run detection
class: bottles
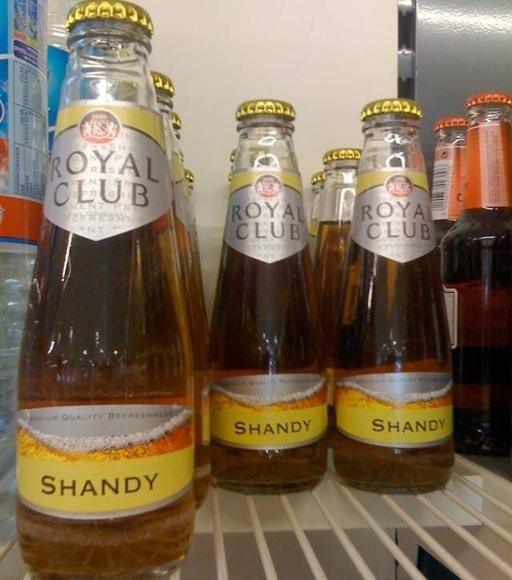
[306,171,328,263]
[148,71,211,515]
[15,1,195,580]
[437,90,512,459]
[328,98,457,495]
[207,98,332,497]
[427,117,471,244]
[309,147,362,450]
